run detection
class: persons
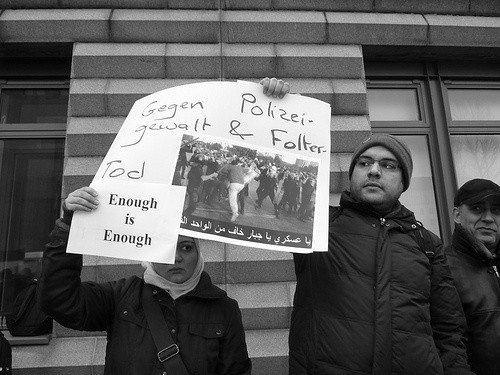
[36,186,252,375]
[172,139,316,222]
[445,179,500,375]
[259,77,471,375]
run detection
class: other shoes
[231,213,239,223]
[254,205,281,220]
[278,206,314,223]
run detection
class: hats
[453,177,500,218]
[349,131,414,192]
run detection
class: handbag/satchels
[6,255,53,336]
